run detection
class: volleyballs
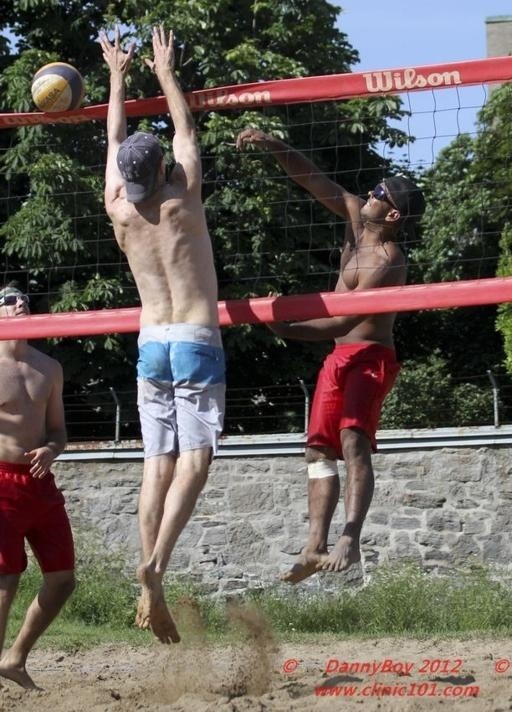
[30,61,85,114]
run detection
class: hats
[384,175,425,225]
[116,133,163,202]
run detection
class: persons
[97,26,226,645]
[0,287,75,688]
[235,129,427,585]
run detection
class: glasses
[374,185,401,211]
[0,295,30,306]
